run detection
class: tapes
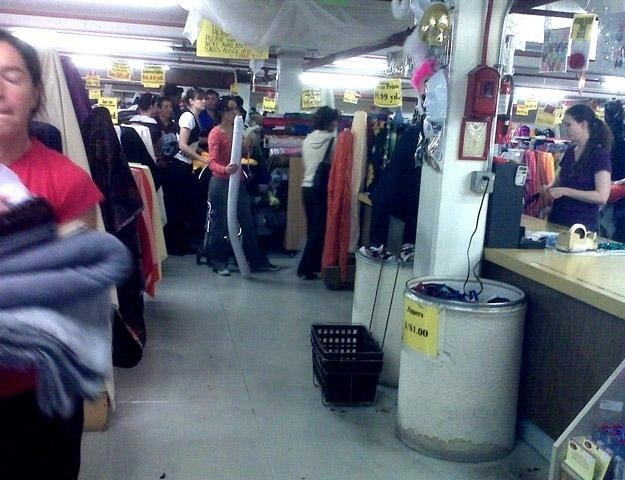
[569,222,591,238]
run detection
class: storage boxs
[310,324,384,407]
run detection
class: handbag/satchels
[159,133,179,162]
[314,137,341,192]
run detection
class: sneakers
[358,243,397,263]
[250,262,281,273]
[399,243,415,263]
[212,265,230,276]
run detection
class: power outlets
[471,170,494,194]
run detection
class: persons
[0,28,105,480]
[160,83,183,99]
[598,180,625,238]
[369,126,426,264]
[177,88,207,246]
[151,97,180,223]
[174,96,187,111]
[520,124,530,137]
[296,105,339,282]
[205,95,281,278]
[128,92,160,140]
[539,105,611,232]
[199,89,223,130]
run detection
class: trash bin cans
[397,274,526,463]
[352,244,424,391]
